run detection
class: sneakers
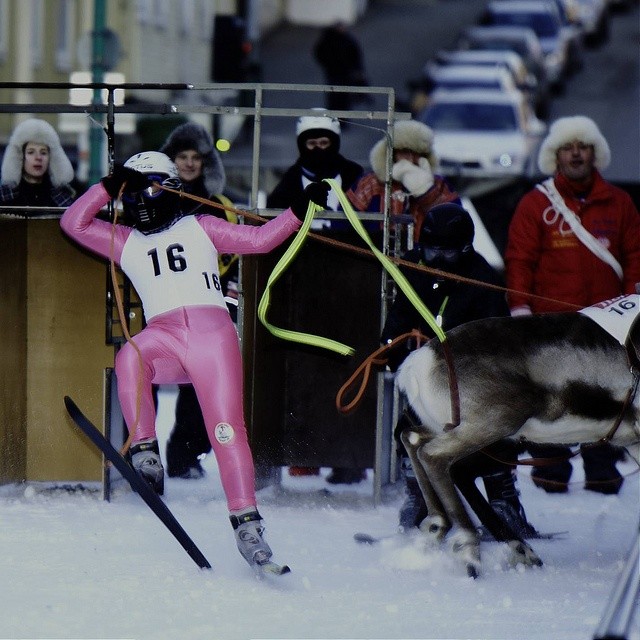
[168,441,207,480]
[289,463,323,478]
[126,438,165,497]
[326,469,368,486]
[228,506,274,567]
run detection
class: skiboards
[64,396,291,575]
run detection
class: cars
[412,0,606,178]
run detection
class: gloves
[0,180,22,205]
[48,182,77,207]
[378,334,409,364]
[99,166,154,200]
[391,158,435,199]
[289,182,332,222]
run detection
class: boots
[527,445,574,495]
[579,443,627,496]
[481,469,537,540]
[400,456,429,532]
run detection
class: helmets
[417,203,477,283]
[120,151,183,233]
[296,108,342,167]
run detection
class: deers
[392,310,640,578]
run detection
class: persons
[405,74,435,120]
[267,107,368,485]
[158,122,240,479]
[338,118,463,249]
[1,118,79,222]
[59,151,331,576]
[505,115,640,496]
[382,202,550,543]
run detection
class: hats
[160,122,226,196]
[370,120,441,184]
[539,116,612,177]
[0,118,74,188]
